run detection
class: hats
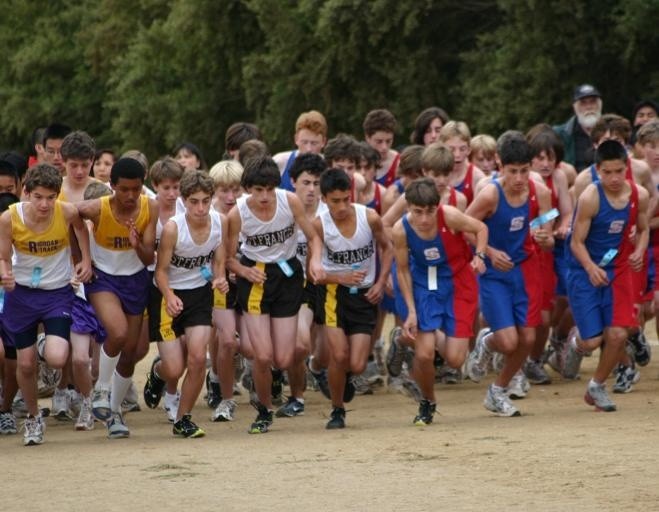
[572,85,599,101]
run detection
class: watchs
[472,252,486,260]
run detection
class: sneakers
[143,357,167,408]
[304,356,331,399]
[276,396,305,417]
[37,333,62,386]
[350,376,374,397]
[23,413,45,445]
[468,328,495,383]
[327,408,346,429]
[508,375,530,397]
[585,379,616,411]
[400,379,422,400]
[444,363,461,384]
[121,401,139,413]
[242,373,262,410]
[205,370,222,408]
[250,406,274,433]
[612,364,640,393]
[492,350,507,374]
[106,411,129,438]
[386,327,409,377]
[75,397,95,428]
[49,394,70,417]
[561,326,592,379]
[174,413,205,439]
[483,385,520,416]
[11,390,27,419]
[414,399,436,426]
[0,411,17,435]
[627,326,651,366]
[522,357,551,384]
[210,400,235,421]
[165,393,180,421]
[90,390,111,419]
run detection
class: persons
[65,156,159,439]
[154,167,232,440]
[558,138,654,414]
[390,179,488,430]
[1,84,658,441]
[1,161,96,448]
[462,135,557,417]
[305,168,394,430]
[225,154,327,436]
